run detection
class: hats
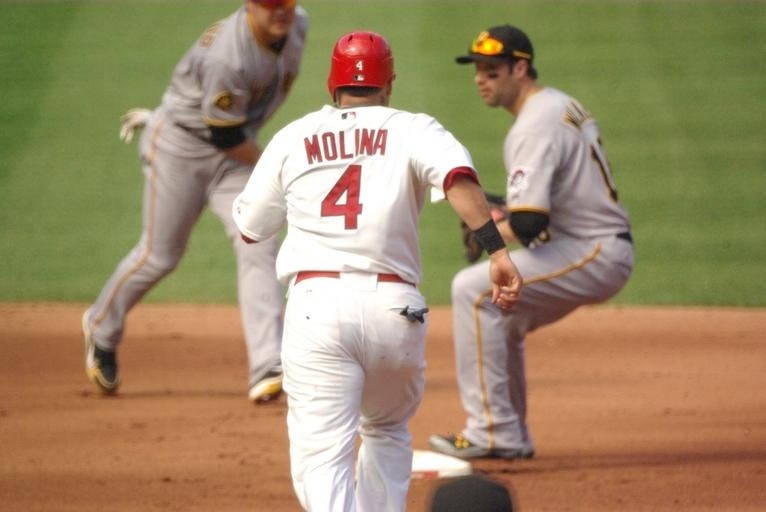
[455,25,533,65]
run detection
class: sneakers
[428,430,534,459]
[86,342,119,392]
[249,363,283,403]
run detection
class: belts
[294,271,415,286]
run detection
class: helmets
[328,32,396,103]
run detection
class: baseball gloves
[459,192,506,261]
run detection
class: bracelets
[474,217,508,257]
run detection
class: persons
[429,23,635,459]
[232,31,526,511]
[80,0,312,403]
